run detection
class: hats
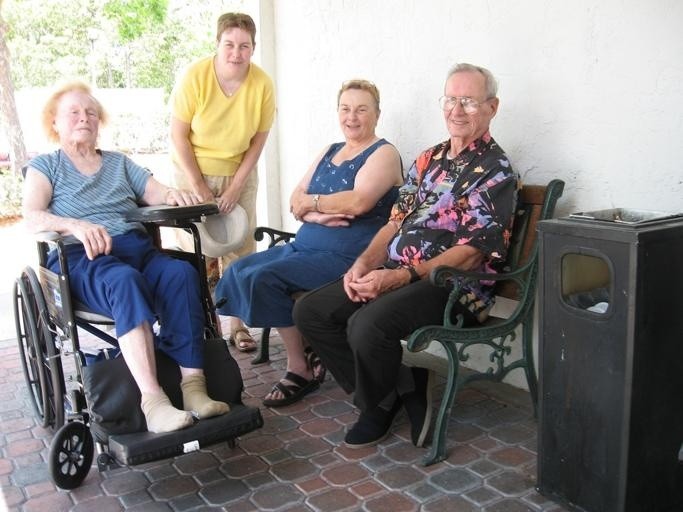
[192,197,249,258]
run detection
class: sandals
[228,326,257,352]
[263,371,319,408]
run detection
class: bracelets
[408,266,421,284]
[312,194,321,212]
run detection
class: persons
[214,80,404,408]
[21,80,230,434]
[291,62,519,448]
[171,13,277,352]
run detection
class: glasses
[438,95,495,115]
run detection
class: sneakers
[343,386,404,449]
[400,363,435,447]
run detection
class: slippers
[303,346,326,384]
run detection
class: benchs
[250,179,565,465]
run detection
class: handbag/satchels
[80,328,162,367]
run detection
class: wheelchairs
[12,200,264,491]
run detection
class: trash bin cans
[535,207,683,512]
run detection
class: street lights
[86,24,98,84]
